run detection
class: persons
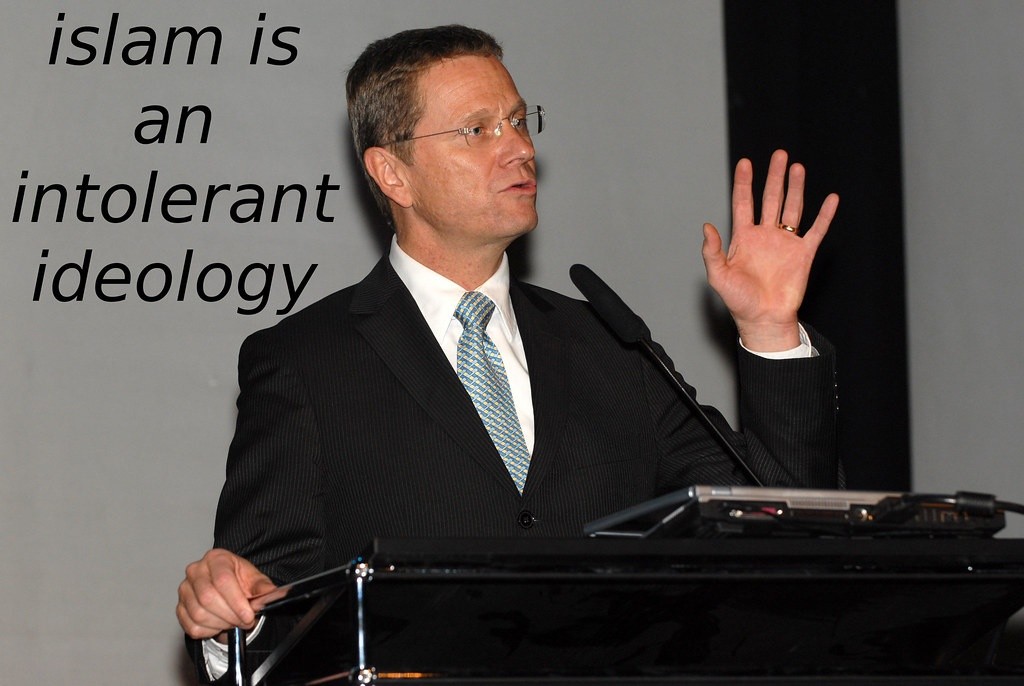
[176,24,841,683]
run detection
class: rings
[779,222,799,235]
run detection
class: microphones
[569,263,766,487]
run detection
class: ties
[452,292,531,497]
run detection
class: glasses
[376,104,549,148]
